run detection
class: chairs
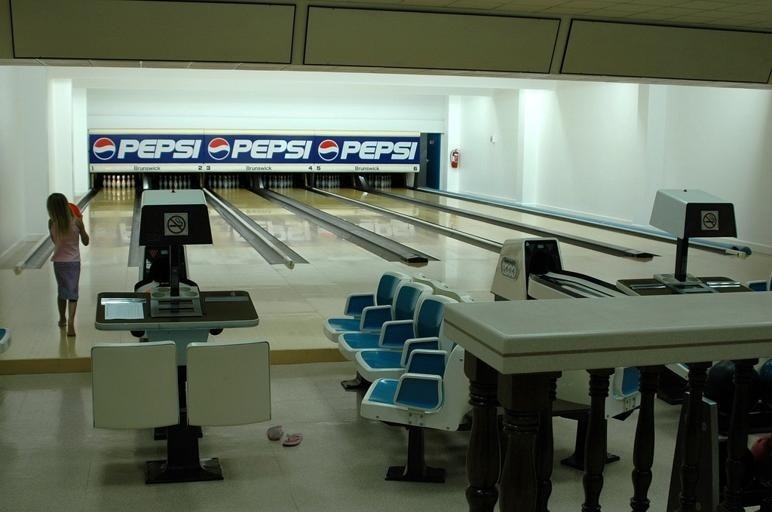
[185,342,272,481]
[360,342,474,482]
[356,295,458,384]
[90,341,177,485]
[337,280,434,361]
[322,270,412,389]
[668,393,728,510]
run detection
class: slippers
[283,432,302,446]
[267,425,283,441]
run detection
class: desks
[445,287,772,512]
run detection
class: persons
[47,193,89,337]
